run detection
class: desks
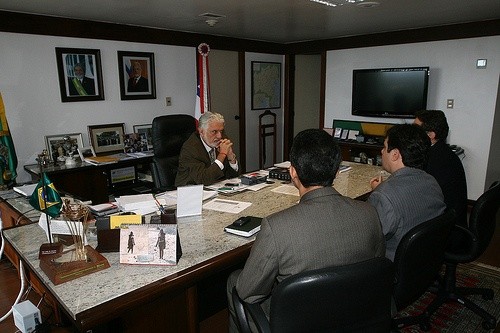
[23,151,153,206]
[0,160,391,333]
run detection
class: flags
[28,173,63,218]
[195,54,210,122]
[0,94,18,190]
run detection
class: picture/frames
[87,121,129,156]
[43,132,85,161]
[55,46,105,104]
[132,123,154,149]
[250,60,282,110]
[116,50,157,101]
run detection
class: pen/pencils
[155,201,164,214]
[156,199,167,214]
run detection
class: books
[225,216,263,237]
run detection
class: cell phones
[234,216,250,227]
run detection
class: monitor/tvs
[351,67,430,118]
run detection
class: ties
[209,149,215,164]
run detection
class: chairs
[417,180,500,330]
[226,255,396,333]
[149,112,201,192]
[392,206,459,314]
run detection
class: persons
[127,62,149,92]
[175,111,239,186]
[370,110,468,228]
[156,229,165,258]
[127,231,135,254]
[97,137,113,146]
[69,63,95,95]
[367,123,446,262]
[124,135,148,153]
[227,127,386,333]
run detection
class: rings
[374,179,378,182]
